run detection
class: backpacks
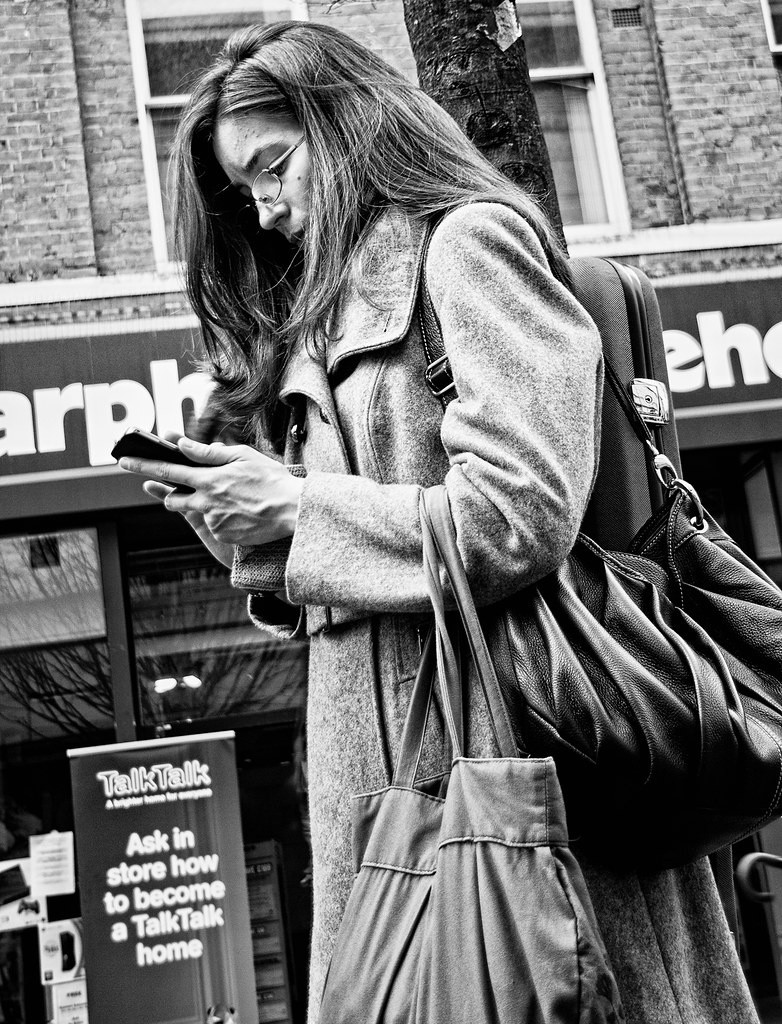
[566,255,685,552]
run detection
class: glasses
[250,131,307,215]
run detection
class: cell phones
[111,426,214,494]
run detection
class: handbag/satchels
[315,480,627,1024]
[417,192,780,879]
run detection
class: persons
[117,21,763,1024]
